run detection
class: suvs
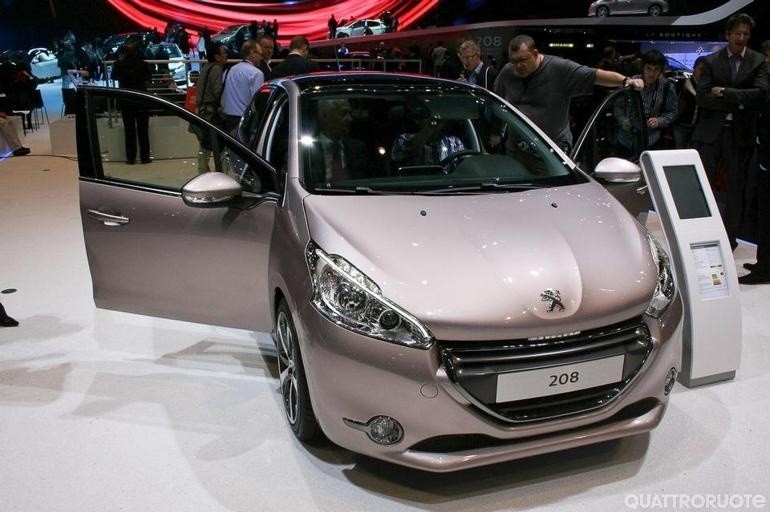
[329,19,386,38]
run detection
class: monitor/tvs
[663,165,713,219]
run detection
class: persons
[2,9,770,285]
[0,301,19,333]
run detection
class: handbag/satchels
[200,116,226,152]
[188,114,221,141]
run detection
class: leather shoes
[0,303,19,327]
[739,274,770,284]
[743,263,756,268]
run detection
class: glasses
[460,52,480,61]
[255,50,265,57]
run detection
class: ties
[332,145,343,182]
[731,55,737,81]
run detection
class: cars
[75,67,688,475]
[0,48,62,81]
[588,1,668,17]
[211,24,264,47]
[103,32,139,57]
[147,44,187,83]
